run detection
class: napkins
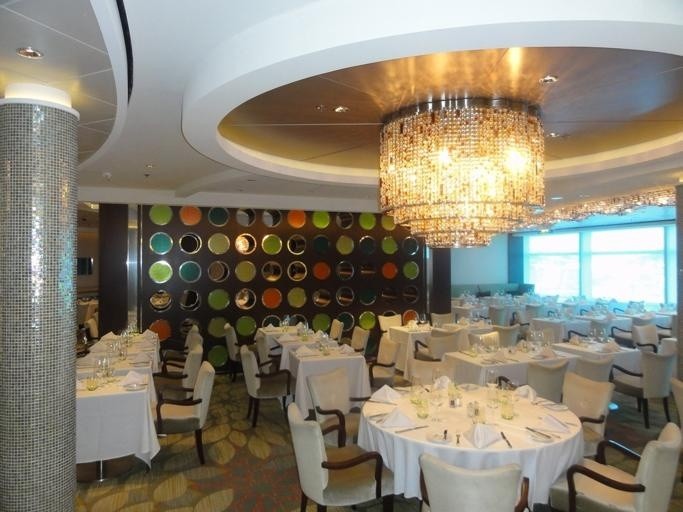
[132,352,150,364]
[368,385,399,404]
[313,330,327,343]
[513,384,538,404]
[296,346,314,356]
[464,423,500,448]
[381,408,412,429]
[339,344,354,355]
[89,341,106,353]
[143,330,154,336]
[120,373,145,385]
[542,414,569,434]
[102,333,116,340]
[137,340,153,349]
[279,334,293,344]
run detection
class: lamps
[377,97,547,255]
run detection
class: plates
[530,432,554,442]
[546,404,568,411]
[126,347,154,391]
[425,432,451,443]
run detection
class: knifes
[442,429,448,440]
[364,398,397,406]
[500,432,512,447]
[395,425,428,434]
[526,427,551,438]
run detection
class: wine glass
[409,369,519,421]
[468,304,610,365]
[85,318,138,391]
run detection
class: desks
[74,332,161,484]
[360,384,583,507]
[258,325,315,371]
[288,347,371,423]
[274,335,339,371]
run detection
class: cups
[412,313,426,325]
[279,319,329,356]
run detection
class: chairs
[224,322,256,380]
[330,318,343,344]
[551,422,682,509]
[240,345,289,428]
[561,373,614,460]
[610,354,675,429]
[306,368,370,443]
[376,282,682,391]
[152,324,203,401]
[286,403,383,510]
[418,453,530,511]
[370,335,398,385]
[156,361,214,466]
[351,327,369,356]
[76,293,99,356]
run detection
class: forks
[455,428,461,444]
